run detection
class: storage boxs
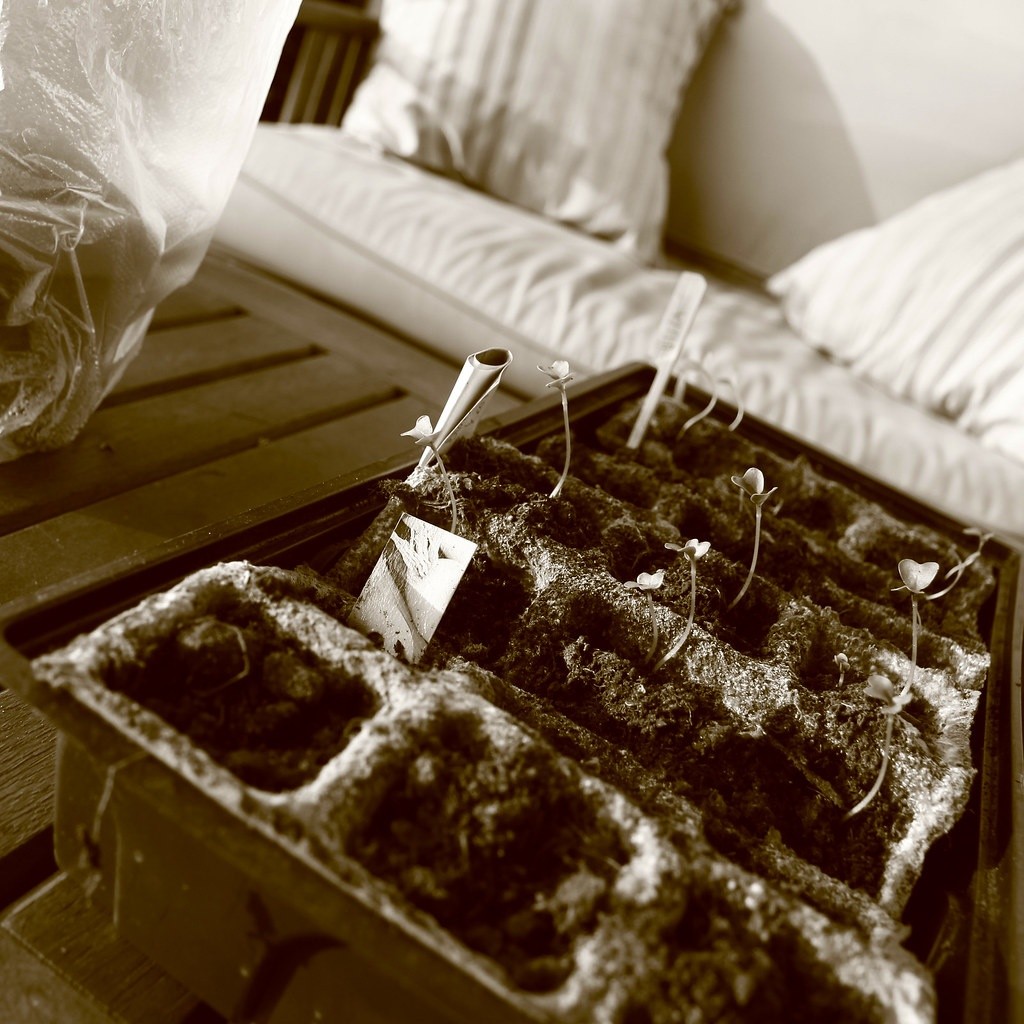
[31,342,1024,1024]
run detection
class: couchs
[198,0,1024,544]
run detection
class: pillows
[336,0,733,259]
[770,159,1024,482]
